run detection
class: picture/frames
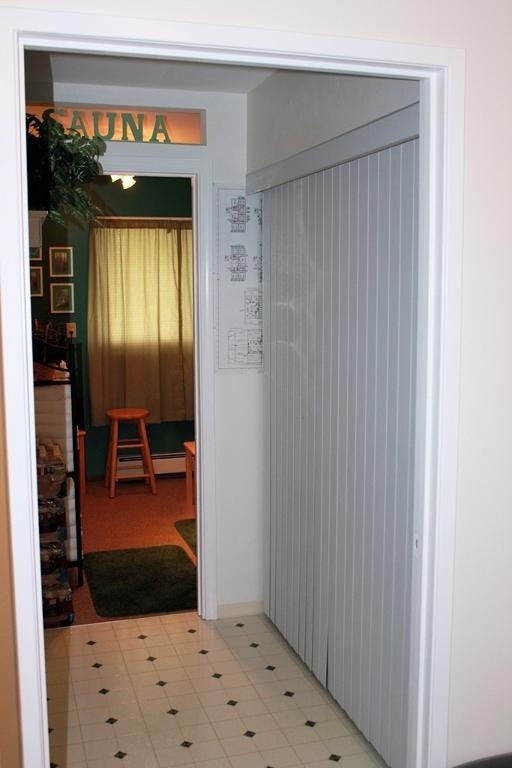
[30,246,76,314]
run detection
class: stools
[103,406,159,499]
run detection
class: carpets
[79,516,201,618]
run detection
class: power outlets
[66,322,76,338]
